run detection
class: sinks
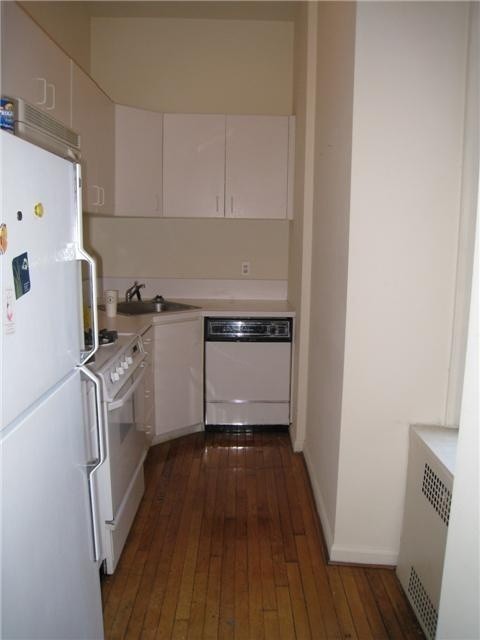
[87,299,203,316]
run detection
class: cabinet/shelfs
[72,62,116,216]
[115,102,162,219]
[162,113,296,221]
[0,0,72,134]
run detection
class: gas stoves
[84,329,147,403]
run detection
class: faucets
[126,281,146,303]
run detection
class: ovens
[101,356,152,577]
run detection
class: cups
[0,99,15,133]
[103,290,118,319]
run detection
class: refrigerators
[0,133,107,640]
